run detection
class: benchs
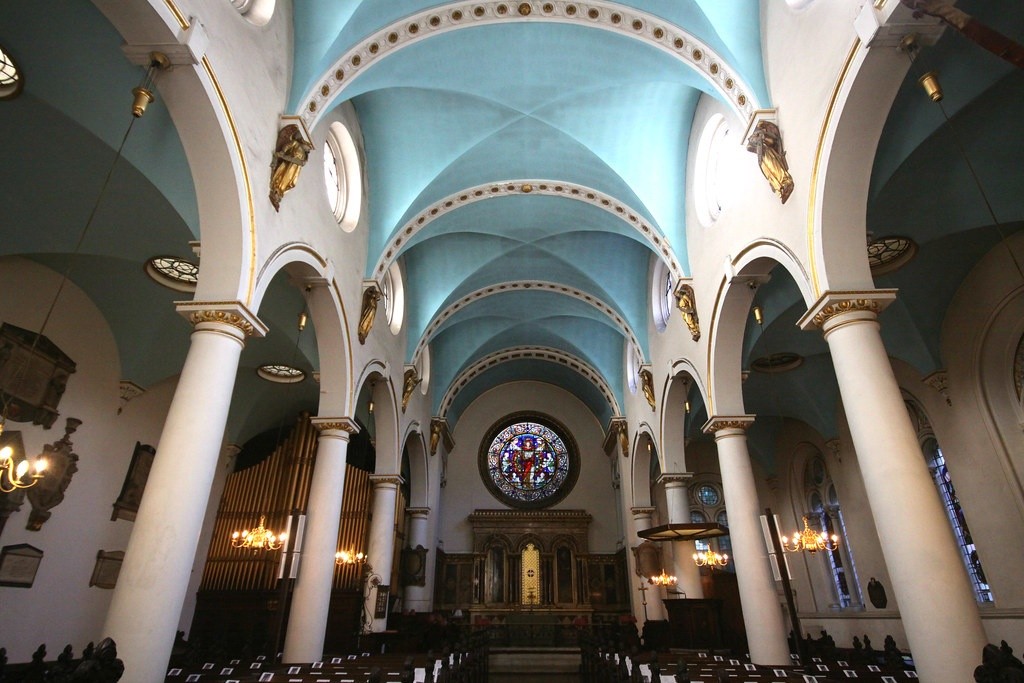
[577,629,922,683]
[0,631,489,683]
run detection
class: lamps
[747,280,838,556]
[335,382,376,566]
[684,381,729,571]
[230,286,311,556]
[0,52,170,493]
[646,438,678,587]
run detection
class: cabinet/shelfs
[661,599,724,651]
